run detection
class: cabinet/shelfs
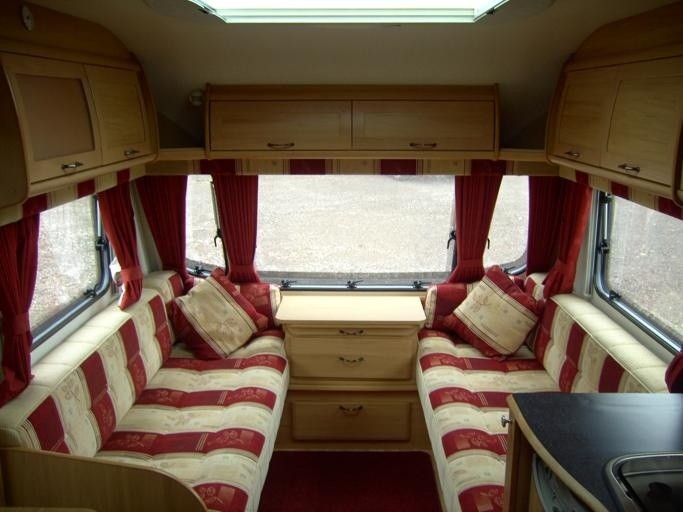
[544,44,683,206]
[203,83,503,161]
[275,296,432,452]
[1,38,159,209]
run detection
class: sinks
[609,452,681,512]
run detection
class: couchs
[414,271,683,512]
[0,269,291,512]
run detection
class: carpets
[256,453,443,512]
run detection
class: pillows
[166,267,269,360]
[440,265,545,364]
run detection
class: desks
[504,393,683,512]
